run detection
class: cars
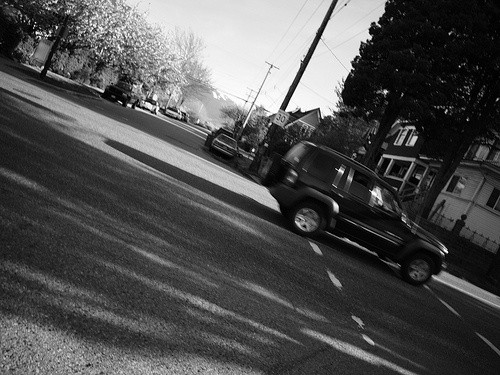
[205,128,235,148]
[209,133,238,160]
[165,107,188,121]
[140,99,160,115]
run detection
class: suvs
[260,139,450,285]
[104,81,141,109]
